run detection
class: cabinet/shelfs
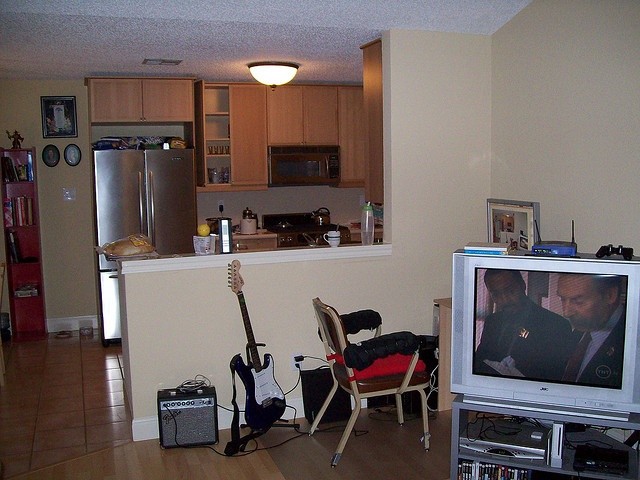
[84,77,195,124]
[215,231,277,254]
[360,38,384,204]
[450,393,639,479]
[183,80,268,192]
[0,146,48,342]
[338,85,364,187]
[268,85,338,187]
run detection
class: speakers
[156,385,219,451]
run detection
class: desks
[433,297,456,413]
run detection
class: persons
[68,147,78,163]
[7,128,25,148]
[542,273,626,388]
[46,149,57,166]
[473,269,576,380]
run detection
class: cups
[323,231,341,247]
[192,235,216,255]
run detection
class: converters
[294,356,305,361]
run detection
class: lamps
[247,62,299,85]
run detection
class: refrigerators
[91,150,197,345]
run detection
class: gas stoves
[268,225,345,233]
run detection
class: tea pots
[310,207,330,228]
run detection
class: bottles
[209,166,229,184]
[361,204,375,246]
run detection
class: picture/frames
[486,198,542,256]
[63,143,81,166]
[42,144,60,167]
[40,96,78,139]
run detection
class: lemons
[198,224,210,236]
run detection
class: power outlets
[289,352,302,371]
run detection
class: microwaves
[270,152,340,184]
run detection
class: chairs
[308,297,430,469]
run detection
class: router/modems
[573,444,629,476]
[531,218,577,256]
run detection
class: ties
[562,332,592,382]
[496,315,516,361]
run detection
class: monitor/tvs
[450,249,640,422]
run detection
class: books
[464,241,511,251]
[8,231,21,263]
[458,461,528,480]
[3,196,33,228]
[28,152,33,181]
[464,247,512,254]
[1,157,19,182]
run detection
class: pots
[206,216,232,234]
